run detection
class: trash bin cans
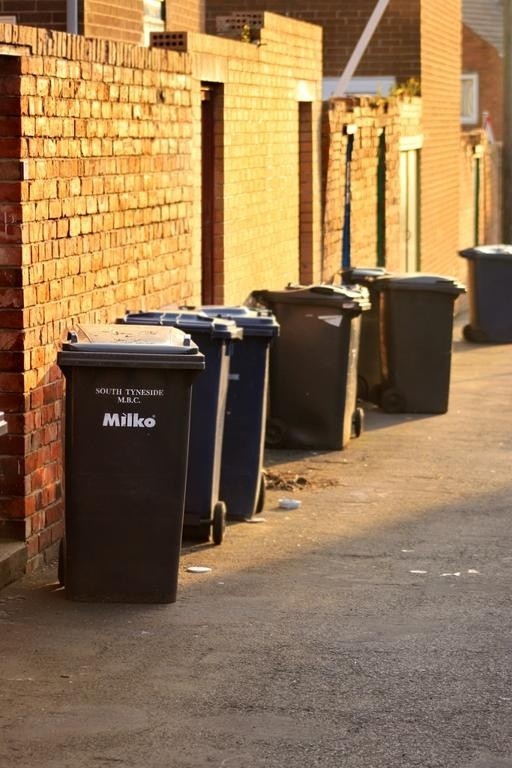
[56,323,206,603]
[190,305,281,521]
[458,243,512,345]
[342,267,390,402]
[250,285,365,451]
[114,308,243,546]
[366,271,469,415]
